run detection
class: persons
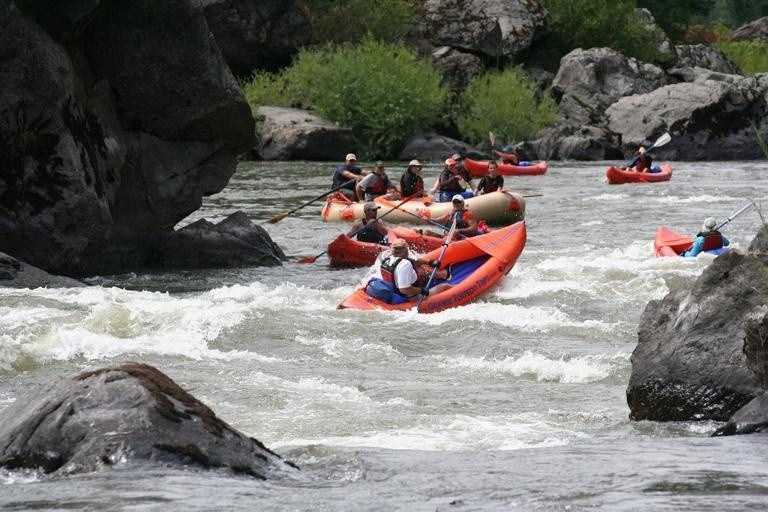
[345,201,390,245]
[472,162,505,196]
[491,144,520,165]
[681,218,733,256]
[427,193,477,241]
[438,158,475,203]
[331,154,371,202]
[356,160,398,203]
[399,158,424,201]
[379,239,453,301]
[628,146,658,174]
[429,154,477,193]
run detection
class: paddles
[299,187,424,264]
[371,197,466,239]
[488,131,495,162]
[620,132,671,169]
[270,180,353,224]
[417,217,458,308]
[678,196,758,257]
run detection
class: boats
[337,219,526,317]
[605,163,674,185]
[654,225,695,259]
[388,225,444,252]
[463,155,548,177]
[321,190,526,227]
[327,233,390,267]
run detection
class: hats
[701,218,718,233]
[346,153,461,169]
[452,194,464,203]
[392,238,410,248]
[364,201,381,210]
